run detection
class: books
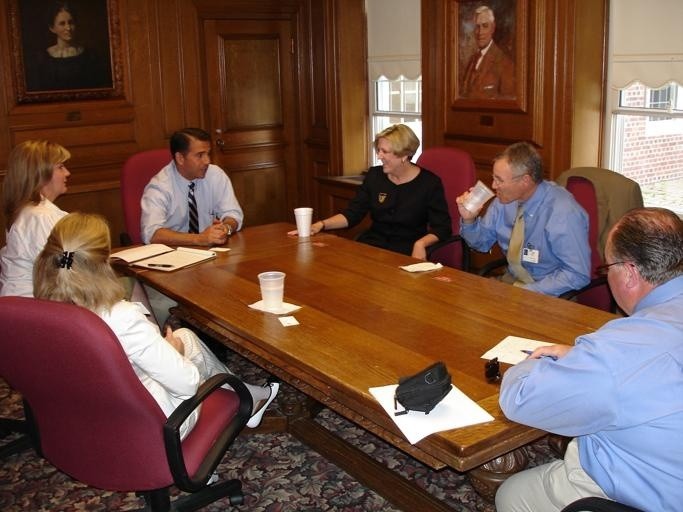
[132,244,220,272]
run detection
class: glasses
[595,261,635,275]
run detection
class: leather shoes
[246,376,280,429]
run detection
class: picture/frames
[6,0,126,105]
[450,0,526,114]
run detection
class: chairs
[474,168,643,314]
[559,495,644,512]
[0,221,40,464]
[0,296,254,511]
[119,149,178,248]
[349,147,476,271]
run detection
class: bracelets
[317,218,329,232]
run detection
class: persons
[460,5,514,97]
[140,127,245,247]
[38,2,102,89]
[0,138,74,298]
[494,207,683,511]
[33,211,280,486]
[455,142,591,298]
[287,124,453,261]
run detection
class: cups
[462,180,495,213]
[293,207,313,238]
[257,271,286,310]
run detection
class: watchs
[225,223,233,236]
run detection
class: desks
[108,221,630,512]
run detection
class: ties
[473,52,482,69]
[187,182,200,234]
[506,206,536,284]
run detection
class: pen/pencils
[148,263,173,267]
[520,349,557,361]
